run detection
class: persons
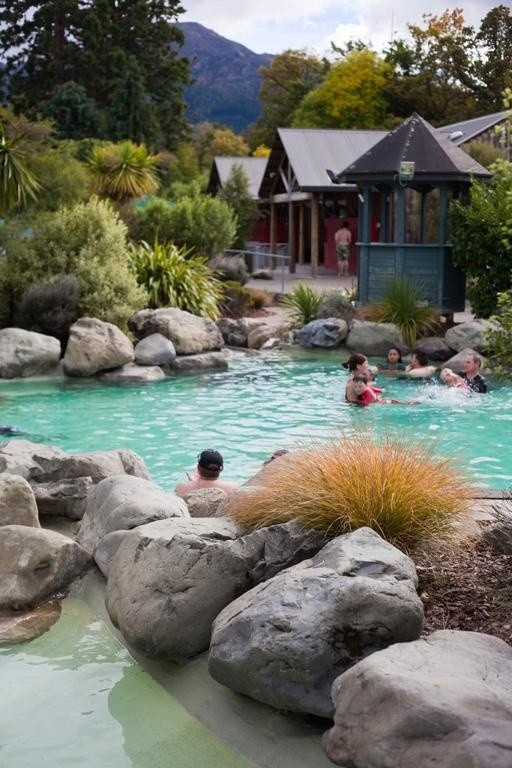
[342,347,487,406]
[335,220,352,278]
[175,448,239,499]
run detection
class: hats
[198,450,222,476]
